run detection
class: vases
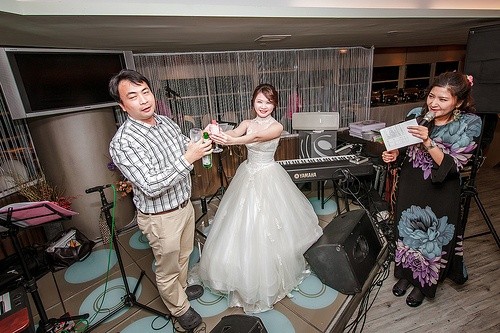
[42,222,64,240]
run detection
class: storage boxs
[349,120,386,134]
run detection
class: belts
[138,200,189,215]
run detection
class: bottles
[201,132,212,169]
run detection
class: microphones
[166,87,180,98]
[418,111,436,127]
[86,184,111,194]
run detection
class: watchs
[425,141,436,150]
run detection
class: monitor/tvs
[0,46,136,119]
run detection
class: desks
[321,129,389,210]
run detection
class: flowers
[0,162,84,211]
[104,154,134,199]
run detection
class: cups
[190,127,203,143]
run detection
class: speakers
[462,24,500,114]
[209,314,268,333]
[302,208,383,295]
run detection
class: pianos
[275,154,374,216]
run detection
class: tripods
[84,188,171,333]
[0,200,89,333]
[206,120,236,204]
[460,113,500,248]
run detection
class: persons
[108,68,214,330]
[382,71,481,307]
[197,84,322,314]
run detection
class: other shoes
[176,306,202,330]
[186,284,204,301]
[392,278,413,297]
[405,287,426,308]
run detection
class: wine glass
[209,125,224,153]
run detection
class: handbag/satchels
[45,226,97,267]
